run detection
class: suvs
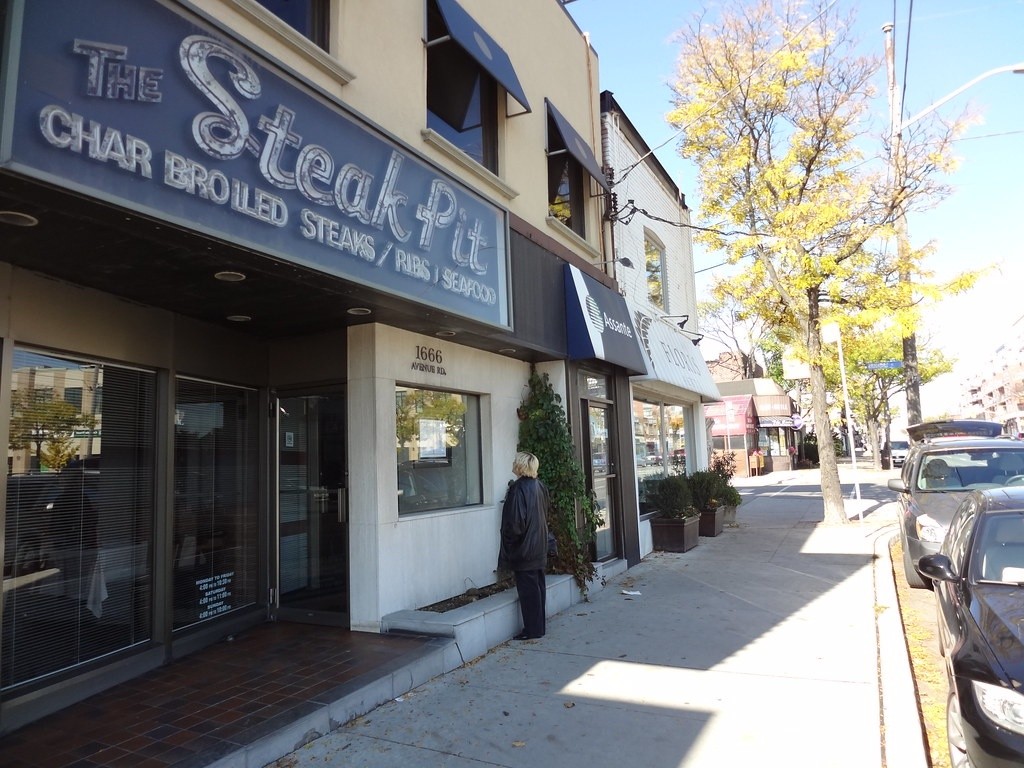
[886,419,1024,589]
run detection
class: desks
[48,538,207,618]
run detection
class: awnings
[547,98,613,196]
[425,0,536,117]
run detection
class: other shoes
[513,633,542,640]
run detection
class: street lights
[821,322,864,521]
[886,60,1023,424]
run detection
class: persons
[50,466,94,552]
[498,450,550,641]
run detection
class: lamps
[681,330,704,345]
[661,314,689,329]
[589,258,635,270]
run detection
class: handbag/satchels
[548,533,559,558]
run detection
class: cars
[914,484,1024,767]
[880,440,910,468]
[6,454,102,577]
[592,449,686,475]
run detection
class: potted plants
[643,471,743,553]
